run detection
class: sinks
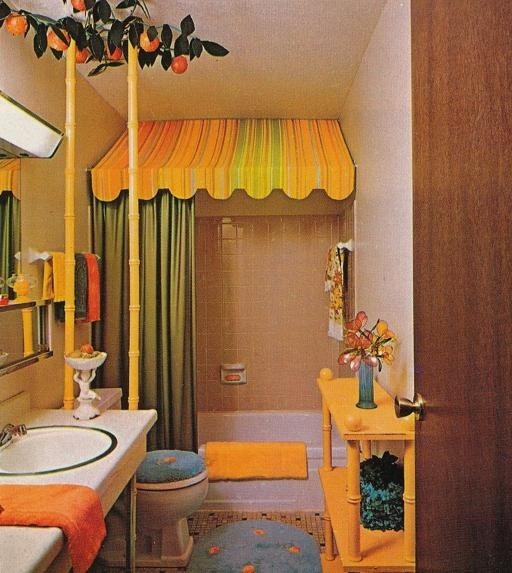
[0,423,120,479]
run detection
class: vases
[355,359,379,410]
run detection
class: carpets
[185,518,322,572]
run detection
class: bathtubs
[196,409,378,512]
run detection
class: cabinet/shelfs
[316,372,416,572]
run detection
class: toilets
[74,386,210,568]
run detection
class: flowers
[333,308,400,374]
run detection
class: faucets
[0,424,27,447]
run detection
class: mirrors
[0,147,24,303]
[0,299,55,376]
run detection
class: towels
[44,250,103,325]
[0,484,109,572]
[204,440,309,482]
